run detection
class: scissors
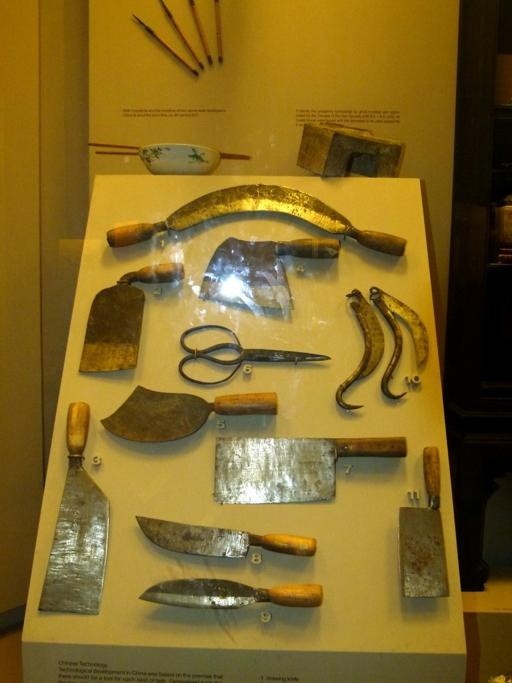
[178,320,331,386]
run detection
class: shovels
[78,260,184,374]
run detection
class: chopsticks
[89,142,253,161]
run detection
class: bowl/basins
[139,142,221,176]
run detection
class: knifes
[213,436,409,506]
[397,446,451,598]
[137,577,324,610]
[38,401,111,615]
[106,183,408,257]
[198,236,342,309]
[134,515,317,559]
